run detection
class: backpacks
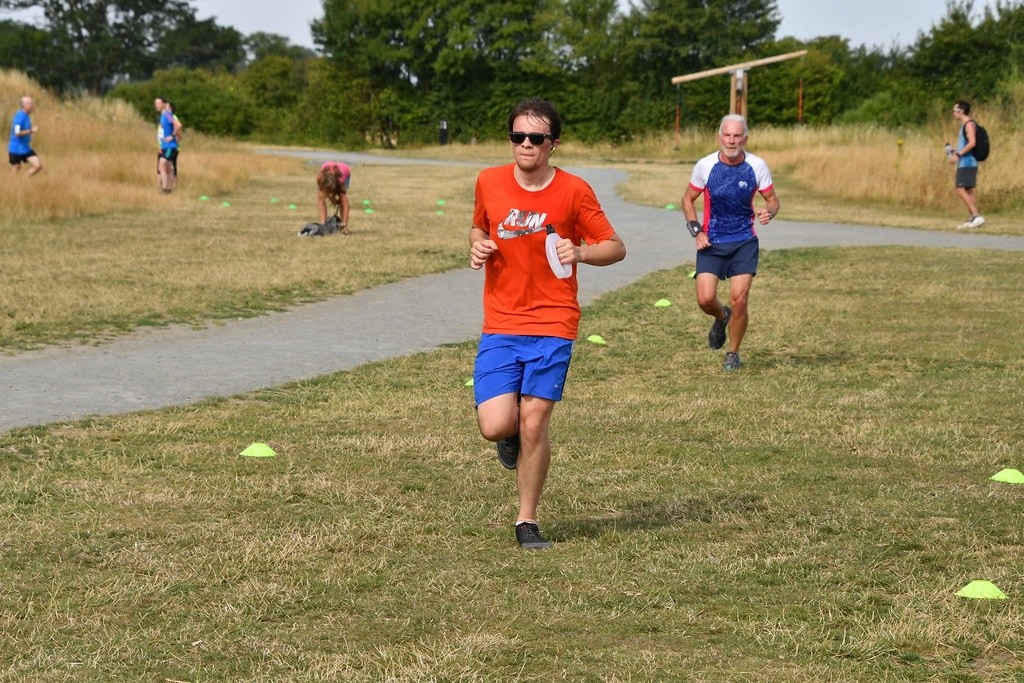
[963,120,990,162]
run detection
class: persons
[8,95,42,177]
[682,114,780,373]
[154,97,181,196]
[468,98,626,548]
[317,161,351,236]
[157,101,181,187]
[945,100,985,230]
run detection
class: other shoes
[968,216,985,227]
[958,221,972,229]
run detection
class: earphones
[549,146,555,157]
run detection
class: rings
[563,254,565,258]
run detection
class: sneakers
[516,522,549,549]
[725,354,739,371]
[496,432,519,469]
[709,306,731,349]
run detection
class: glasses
[509,132,552,146]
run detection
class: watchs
[769,210,774,220]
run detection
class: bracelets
[956,152,961,158]
[29,129,31,132]
[171,133,175,138]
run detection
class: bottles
[944,143,953,158]
[545,224,572,279]
